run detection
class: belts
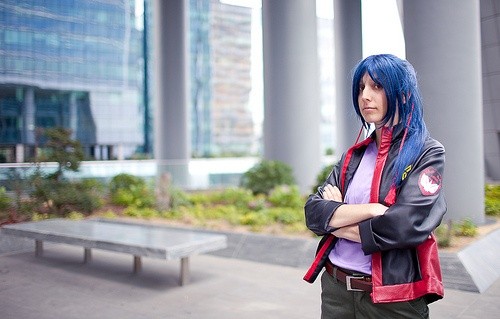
[323,261,373,292]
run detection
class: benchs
[1,216,230,287]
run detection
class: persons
[304,54,449,319]
